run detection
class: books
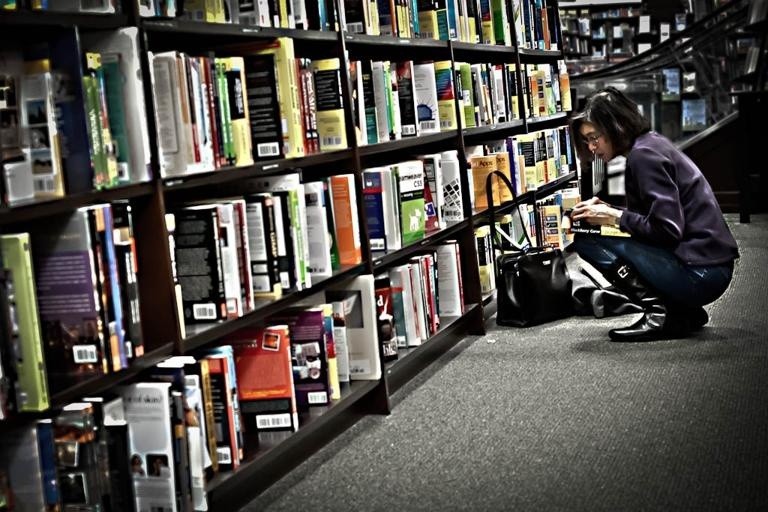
[591,5,766,131]
[1,127,582,511]
[560,207,631,238]
[0,0,588,210]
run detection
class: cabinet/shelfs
[338,0,487,416]
[509,1,589,246]
[130,0,390,509]
[560,1,718,200]
[2,1,190,507]
[445,0,541,333]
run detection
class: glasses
[580,130,606,149]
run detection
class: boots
[601,255,710,343]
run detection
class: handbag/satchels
[493,244,574,329]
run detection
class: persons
[566,85,740,342]
